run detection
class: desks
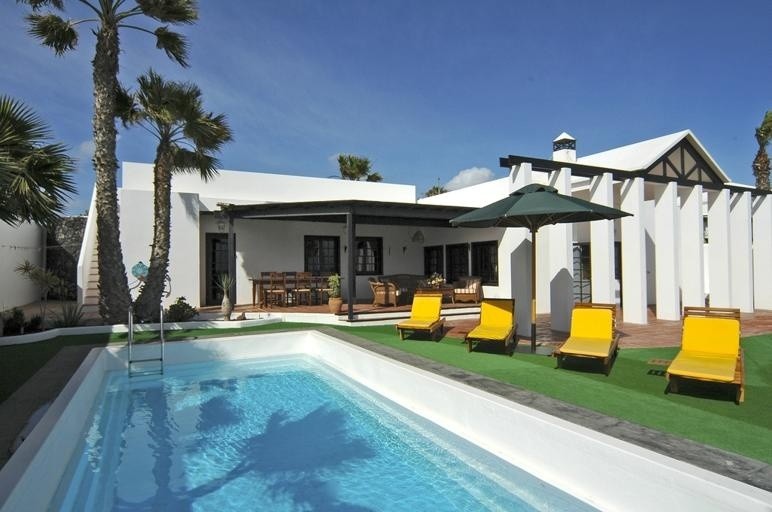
[412,287,454,304]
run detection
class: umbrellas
[450,180,635,353]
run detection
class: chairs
[369,277,401,308]
[454,277,480,304]
[555,301,621,374]
[667,306,746,406]
[466,298,520,356]
[396,288,446,341]
[250,272,338,309]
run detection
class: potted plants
[213,273,237,321]
[326,275,343,313]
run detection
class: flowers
[426,272,446,285]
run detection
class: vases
[432,283,440,289]
